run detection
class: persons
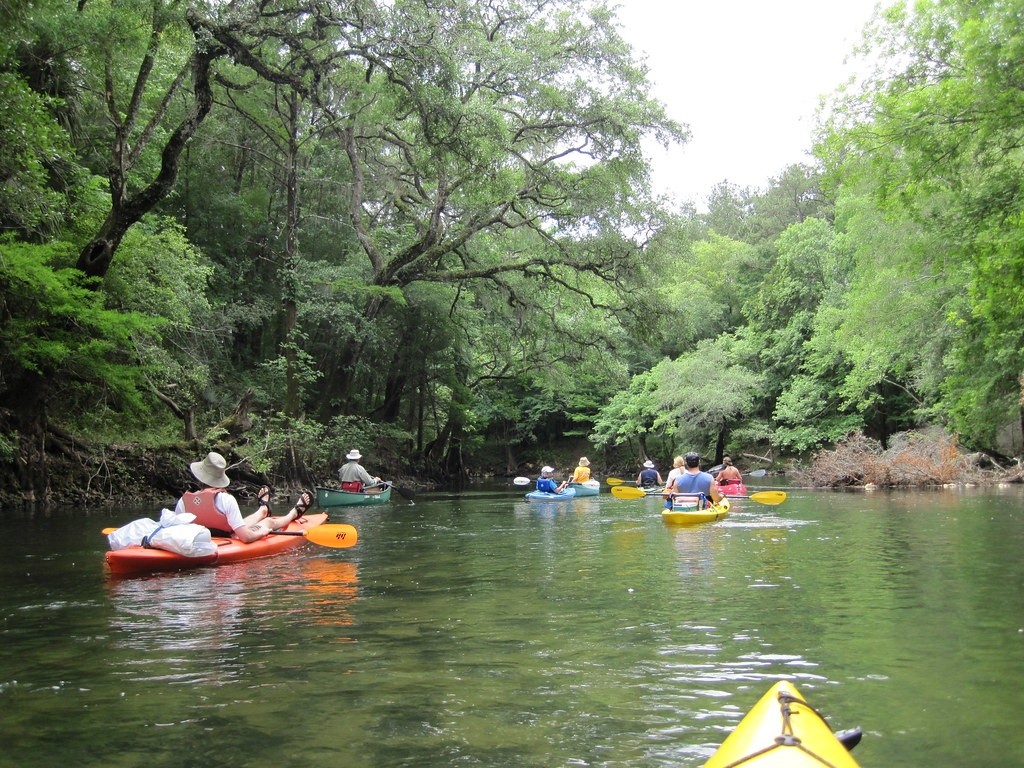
[674,452,725,503]
[716,457,743,486]
[665,455,687,488]
[174,452,315,543]
[338,450,381,492]
[536,466,569,493]
[571,457,590,483]
[635,460,665,488]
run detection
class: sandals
[290,491,314,520]
[256,486,272,519]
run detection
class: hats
[724,457,732,465]
[685,451,699,464]
[190,452,230,488]
[643,460,655,469]
[541,465,554,474]
[579,456,589,467]
[347,450,362,460]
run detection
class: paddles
[611,485,787,506]
[368,475,415,500]
[606,478,668,486]
[513,477,600,489]
[102,524,358,548]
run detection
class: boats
[660,492,732,525]
[715,478,751,497]
[525,478,603,507]
[638,483,663,496]
[105,512,325,581]
[314,479,399,511]
